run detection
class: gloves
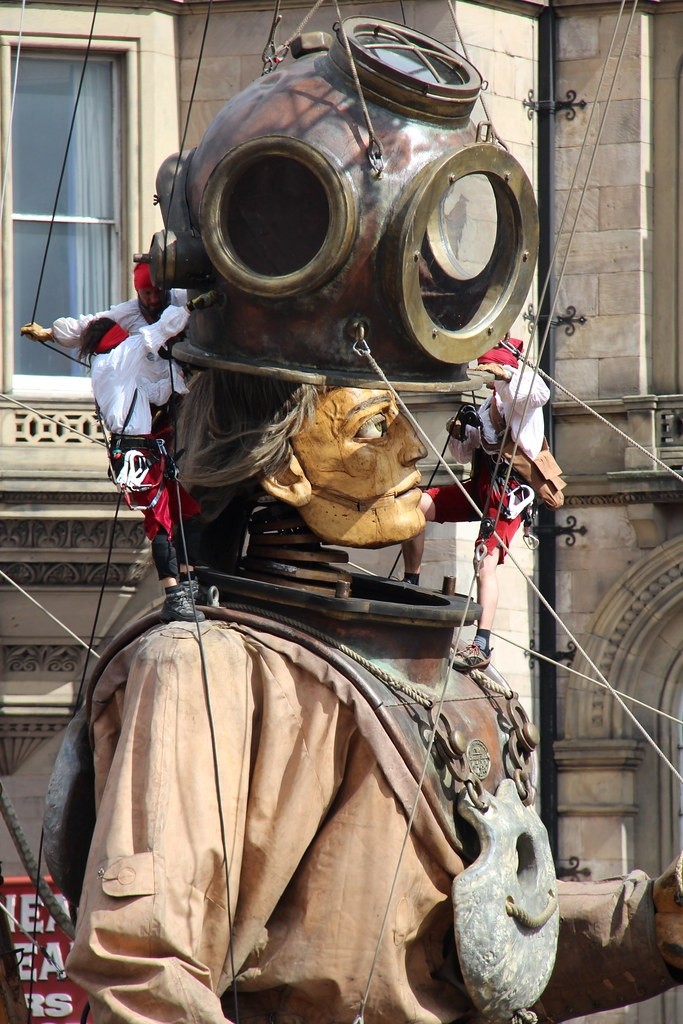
[445,416,462,439]
[23,322,56,343]
[186,293,211,310]
[476,364,509,389]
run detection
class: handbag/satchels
[504,437,567,510]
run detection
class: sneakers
[180,580,199,603]
[159,590,205,622]
[448,642,494,674]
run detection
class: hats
[479,336,524,369]
[133,262,154,291]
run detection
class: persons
[402,339,550,673]
[83,290,219,622]
[41,370,682,1024]
[21,262,189,408]
